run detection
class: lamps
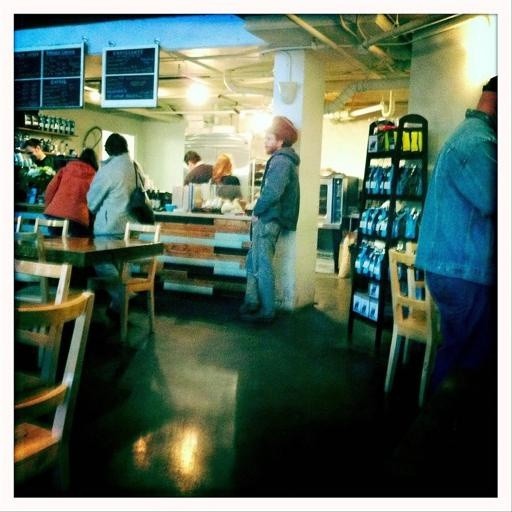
[15,226,63,366]
[398,240,423,368]
[33,210,72,240]
[88,219,164,342]
[381,246,445,405]
[14,255,74,398]
[14,288,95,481]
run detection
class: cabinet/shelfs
[354,245,403,283]
[367,163,420,197]
[24,114,76,135]
[15,133,70,157]
[148,189,169,210]
[359,204,417,238]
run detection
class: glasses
[278,77,299,104]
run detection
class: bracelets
[165,204,176,213]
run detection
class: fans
[101,45,159,108]
[14,43,84,109]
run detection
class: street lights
[130,161,154,225]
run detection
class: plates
[342,112,431,343]
[14,121,84,159]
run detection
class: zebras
[316,174,359,274]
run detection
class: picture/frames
[28,236,168,359]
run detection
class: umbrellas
[240,302,259,314]
[244,313,273,323]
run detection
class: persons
[42,148,99,239]
[412,75,496,407]
[237,114,301,323]
[22,138,55,170]
[85,132,146,319]
[183,151,213,184]
[208,153,242,202]
